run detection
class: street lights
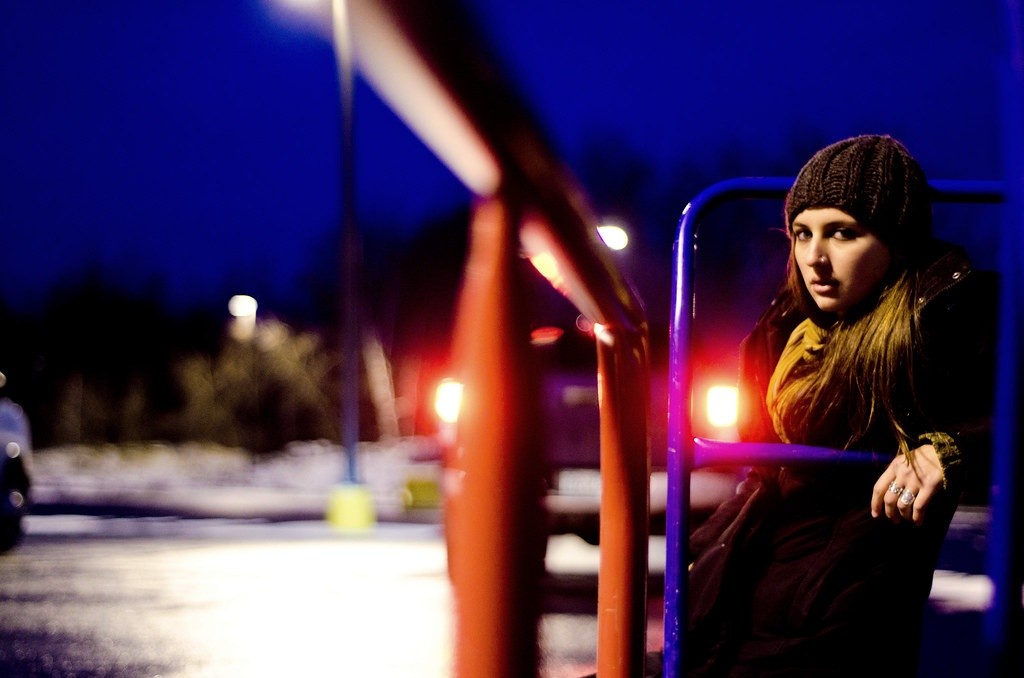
[229,293,258,460]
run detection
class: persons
[673,136,996,677]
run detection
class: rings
[888,481,903,495]
[900,489,915,505]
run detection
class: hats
[787,134,932,266]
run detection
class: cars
[417,328,749,480]
